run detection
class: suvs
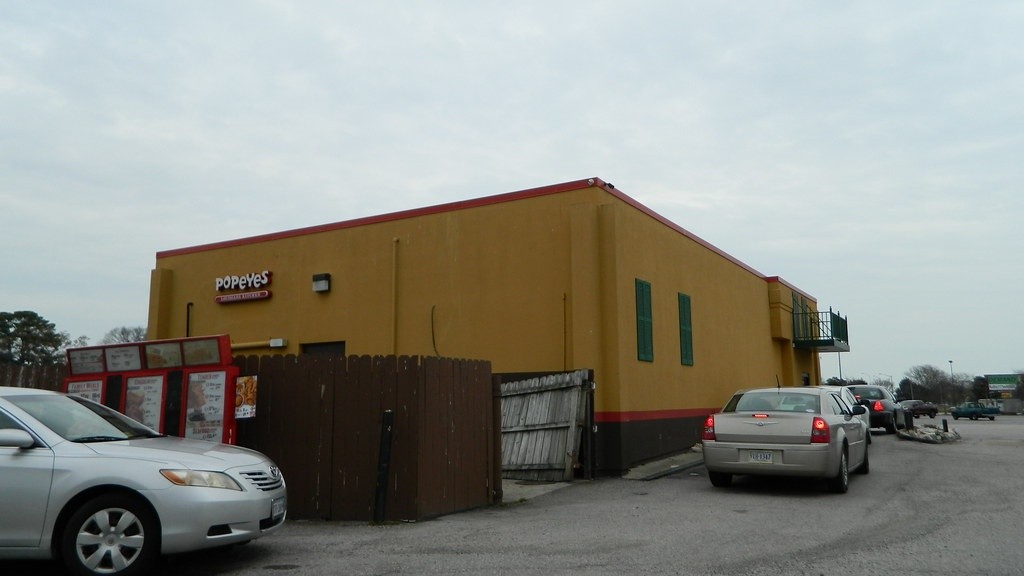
[899,400,939,419]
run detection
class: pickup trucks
[949,402,1001,420]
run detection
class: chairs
[746,397,776,412]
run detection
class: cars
[846,385,909,434]
[0,387,287,576]
[701,375,872,494]
[807,385,871,431]
